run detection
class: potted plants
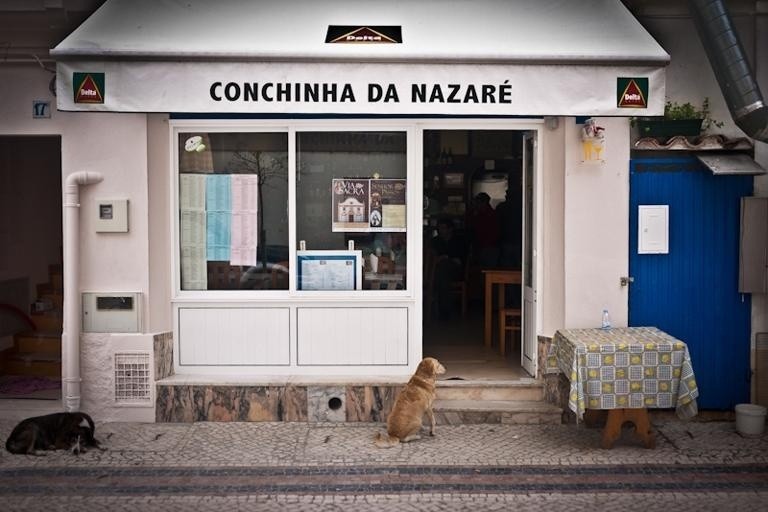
[629,96,725,138]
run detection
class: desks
[481,267,521,349]
[542,326,699,451]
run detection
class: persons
[431,192,507,322]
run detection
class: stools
[498,307,521,356]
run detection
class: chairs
[448,249,471,322]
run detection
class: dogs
[5,411,97,456]
[373,357,448,450]
